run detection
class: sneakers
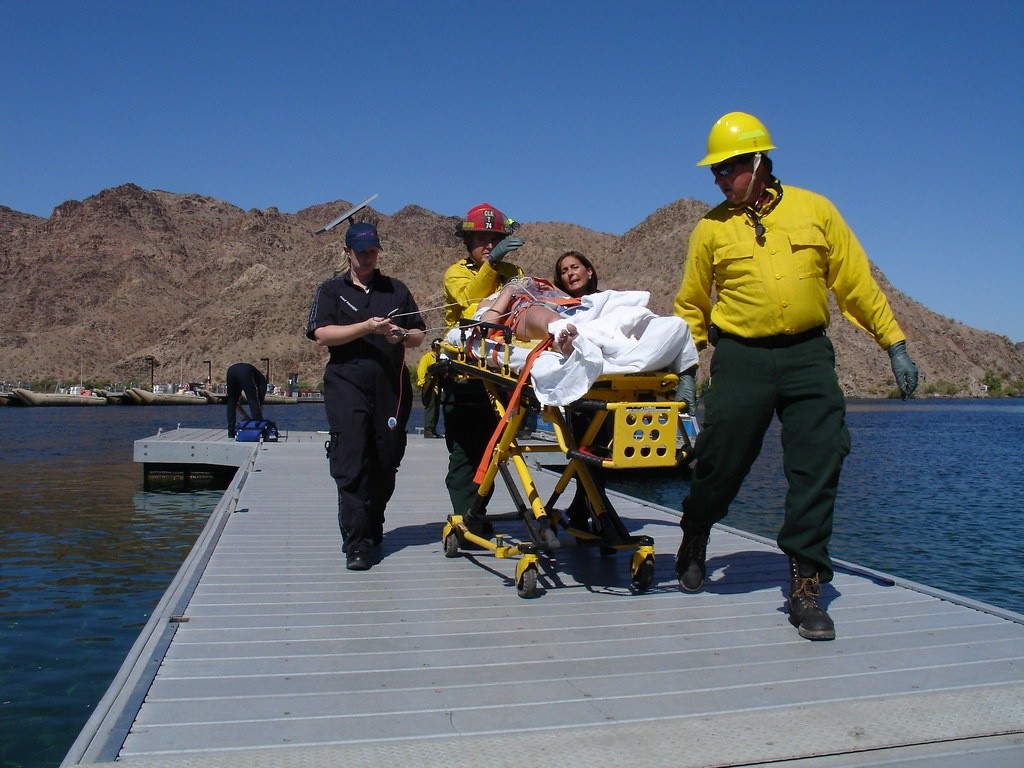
[676,530,709,592]
[789,558,836,640]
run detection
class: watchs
[401,328,409,344]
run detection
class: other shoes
[372,522,383,546]
[346,543,370,570]
[483,521,493,534]
[424,432,443,439]
[569,517,597,547]
[593,525,617,557]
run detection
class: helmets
[462,203,511,235]
[696,112,777,166]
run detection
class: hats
[345,221,383,253]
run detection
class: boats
[91,388,130,405]
[126,388,208,405]
[11,388,107,406]
[197,388,297,404]
[0,395,27,407]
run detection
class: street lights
[145,356,153,392]
[261,357,270,385]
[203,360,212,384]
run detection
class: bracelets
[488,308,502,316]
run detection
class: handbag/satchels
[236,419,278,442]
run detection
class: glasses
[710,154,755,178]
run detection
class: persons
[226,362,267,438]
[306,222,426,570]
[478,276,590,360]
[441,202,526,550]
[415,338,449,438]
[674,112,920,639]
[555,250,617,558]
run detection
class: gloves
[674,367,699,416]
[888,345,918,401]
[489,236,526,263]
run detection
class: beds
[432,317,680,404]
